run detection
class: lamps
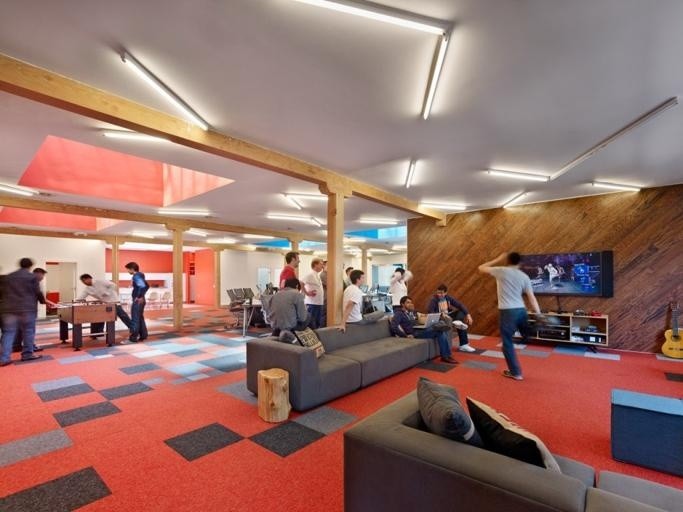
[309,3,449,34]
[0,184,34,199]
[422,35,450,121]
[284,194,329,201]
[487,167,550,185]
[359,220,400,226]
[288,200,304,212]
[593,181,643,194]
[158,209,213,218]
[122,51,210,131]
[405,161,417,190]
[267,215,324,226]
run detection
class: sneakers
[503,370,522,379]
[459,344,476,352]
[120,340,136,345]
[451,320,468,330]
[33,346,43,352]
[537,314,558,325]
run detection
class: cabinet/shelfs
[509,312,608,346]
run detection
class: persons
[12,268,55,352]
[390,268,414,312]
[478,251,540,380]
[269,253,364,335]
[427,284,476,353]
[120,262,149,345]
[389,296,458,364]
[76,275,132,337]
[544,263,560,288]
[0,259,45,366]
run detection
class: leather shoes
[0,361,13,366]
[431,322,451,332]
[441,356,457,363]
[21,352,41,361]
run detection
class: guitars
[662,302,683,359]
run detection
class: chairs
[127,291,172,311]
[227,282,394,337]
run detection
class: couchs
[244,312,453,412]
[341,375,682,511]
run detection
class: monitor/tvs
[510,252,615,297]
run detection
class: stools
[610,387,683,479]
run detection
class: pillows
[415,376,484,449]
[464,393,563,474]
[292,326,328,360]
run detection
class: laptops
[413,312,442,329]
